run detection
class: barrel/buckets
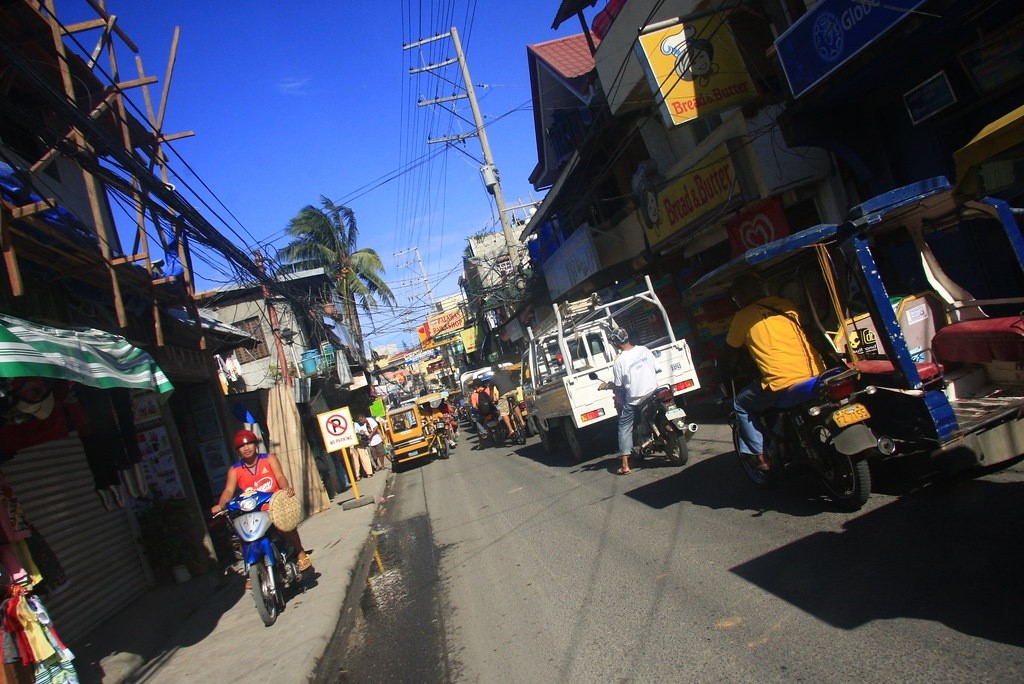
[301,349,321,375]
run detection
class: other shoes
[450,441,455,446]
[508,430,514,439]
[521,422,526,428]
[455,432,460,437]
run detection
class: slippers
[617,467,631,475]
[755,460,770,470]
[296,553,312,571]
[245,578,252,589]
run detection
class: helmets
[491,364,498,370]
[234,429,258,450]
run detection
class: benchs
[847,358,944,388]
[931,316,1024,364]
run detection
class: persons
[725,272,832,473]
[211,429,312,593]
[346,362,527,481]
[598,326,663,475]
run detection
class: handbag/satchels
[356,431,369,448]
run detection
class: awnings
[0,312,175,412]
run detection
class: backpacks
[475,387,494,415]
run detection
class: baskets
[268,489,301,531]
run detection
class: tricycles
[690,174,1024,486]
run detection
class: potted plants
[319,281,335,314]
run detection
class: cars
[418,392,459,439]
[521,274,702,460]
[457,362,543,439]
[385,403,434,469]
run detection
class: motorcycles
[712,348,879,508]
[586,367,697,468]
[428,417,451,459]
[211,491,292,626]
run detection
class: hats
[608,327,628,343]
